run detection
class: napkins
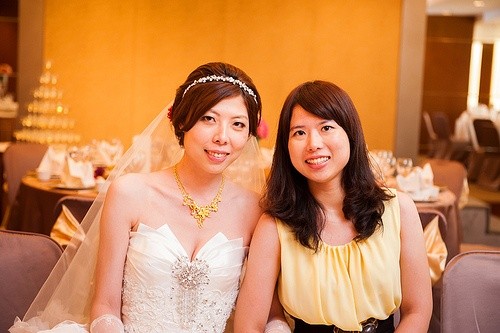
[60,156,94,188]
[36,146,65,180]
[396,162,440,200]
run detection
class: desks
[8,165,461,333]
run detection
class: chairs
[439,250,500,333]
[50,196,95,250]
[0,229,68,333]
[423,110,500,207]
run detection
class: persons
[90,61,291,333]
[232,80,433,333]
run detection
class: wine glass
[368,150,413,196]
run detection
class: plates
[413,199,439,203]
[50,184,96,189]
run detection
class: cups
[99,139,123,170]
[49,144,67,174]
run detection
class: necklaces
[174,164,224,229]
